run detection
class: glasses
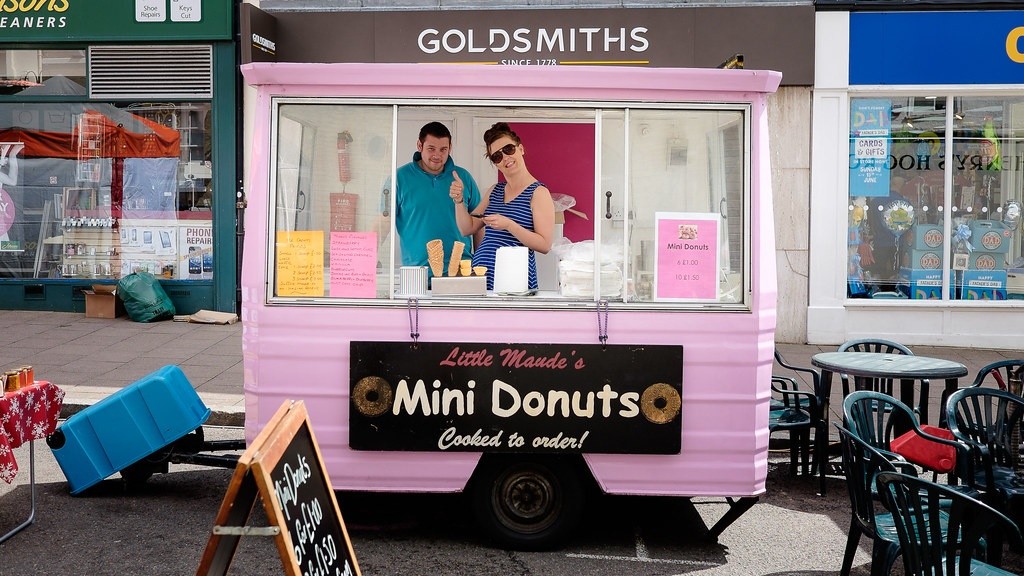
[490,144,519,164]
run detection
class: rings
[490,224,492,226]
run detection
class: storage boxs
[492,246,530,293]
[970,252,1007,270]
[965,221,1012,253]
[1,240,25,250]
[45,360,211,498]
[431,276,488,298]
[956,270,1007,300]
[901,223,943,250]
[896,269,957,298]
[897,249,942,269]
[953,253,969,270]
[80,283,121,319]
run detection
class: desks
[807,350,971,487]
[0,377,63,543]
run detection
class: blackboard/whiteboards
[196,399,362,576]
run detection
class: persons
[0,145,24,242]
[372,122,485,290]
[450,122,555,288]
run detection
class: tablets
[121,228,171,248]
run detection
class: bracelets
[453,198,463,203]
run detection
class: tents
[0,75,181,277]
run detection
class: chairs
[840,391,991,506]
[947,387,1024,507]
[832,423,988,576]
[874,471,1024,576]
[768,348,822,470]
[937,355,1024,446]
[769,377,820,482]
[838,337,932,449]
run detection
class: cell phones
[189,246,215,274]
